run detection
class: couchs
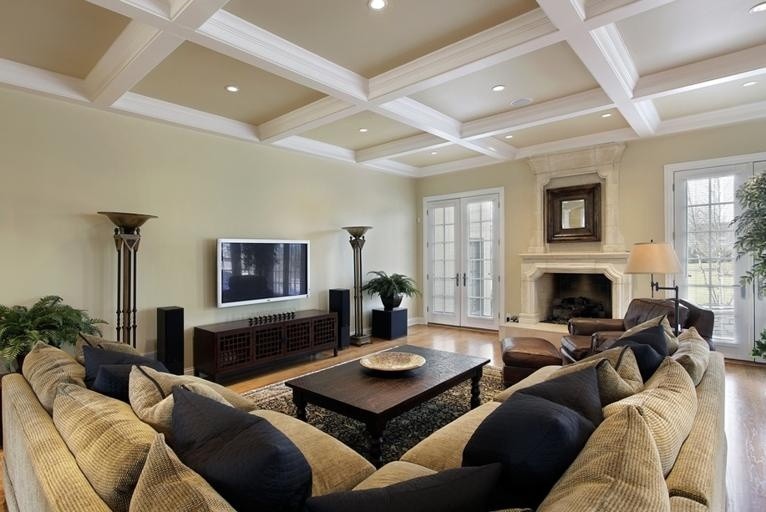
[1,325,723,512]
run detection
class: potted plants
[356,265,420,309]
[0,292,110,375]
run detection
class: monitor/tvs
[217,237,311,309]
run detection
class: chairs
[559,296,714,363]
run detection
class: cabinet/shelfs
[193,307,340,387]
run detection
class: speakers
[329,288,350,351]
[156,305,185,376]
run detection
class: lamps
[339,225,376,346]
[98,209,158,350]
[621,238,683,336]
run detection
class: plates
[360,352,426,374]
